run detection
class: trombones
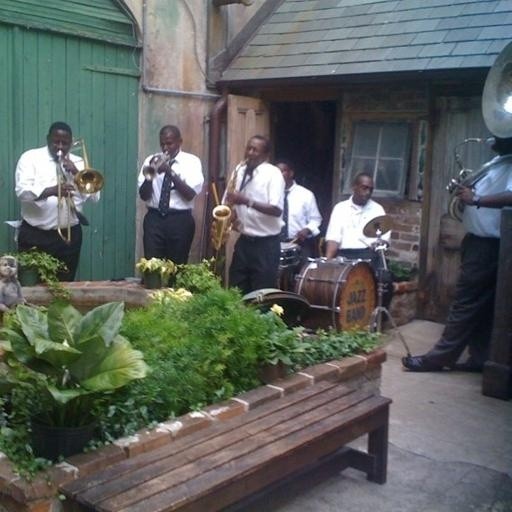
[57,140,103,244]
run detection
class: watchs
[246,199,256,207]
[471,195,480,209]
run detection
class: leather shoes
[450,357,483,373]
[402,356,440,372]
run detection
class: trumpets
[143,149,171,182]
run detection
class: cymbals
[364,215,392,237]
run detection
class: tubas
[448,41,512,222]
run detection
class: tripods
[369,231,411,358]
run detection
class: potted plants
[255,330,312,382]
[136,257,177,290]
[17,249,72,298]
[0,302,151,464]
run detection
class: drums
[295,255,378,332]
[278,241,299,288]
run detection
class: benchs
[59,381,394,512]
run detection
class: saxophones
[211,158,248,251]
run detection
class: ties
[282,191,288,241]
[157,160,175,217]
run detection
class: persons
[0,255,26,312]
[14,122,100,287]
[274,159,323,272]
[402,138,512,372]
[137,125,204,289]
[324,173,393,332]
[221,135,286,297]
